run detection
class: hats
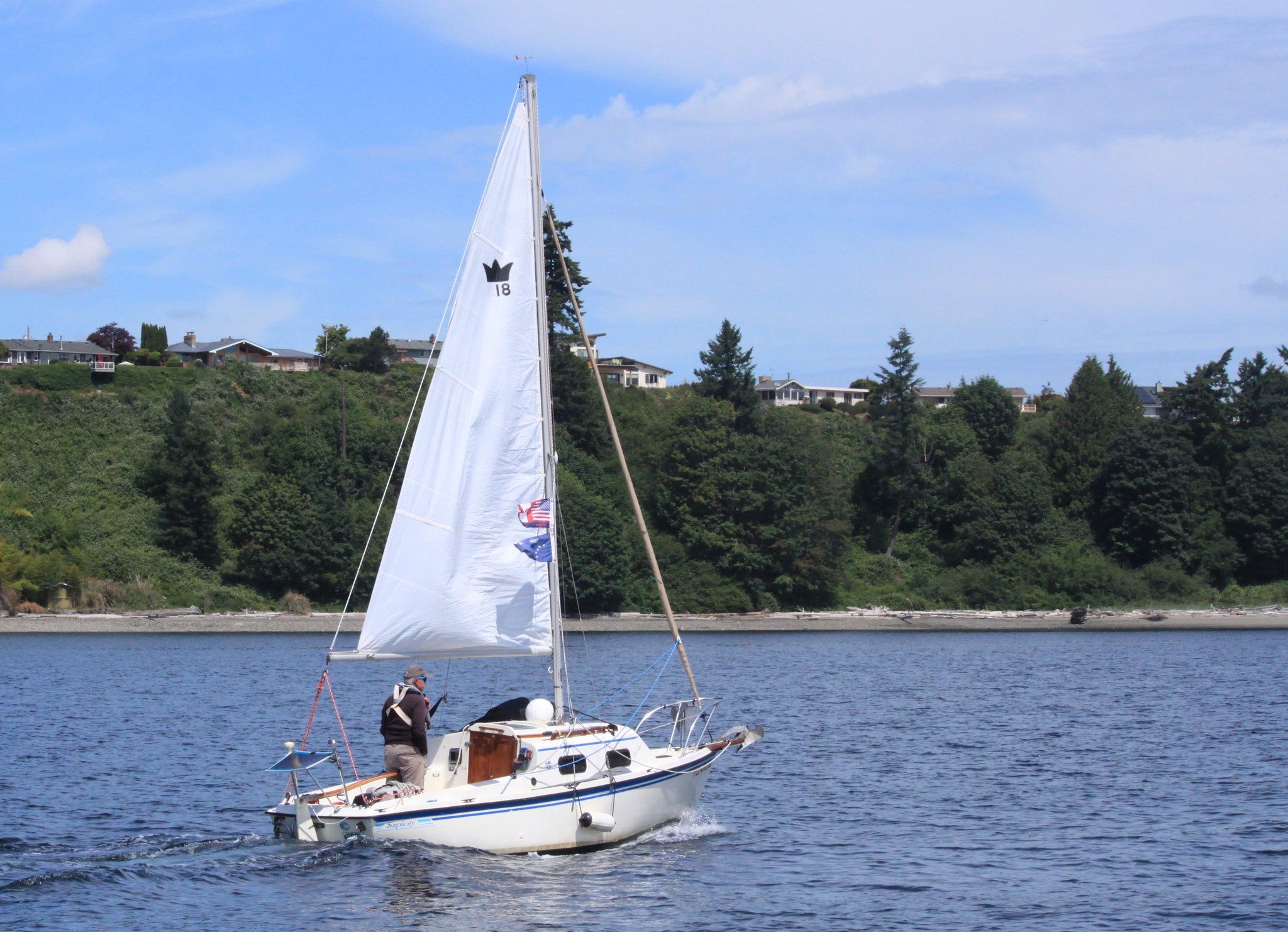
[404,664,432,678]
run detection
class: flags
[518,498,554,529]
[514,532,556,563]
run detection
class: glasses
[413,677,427,682]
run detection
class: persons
[380,664,433,794]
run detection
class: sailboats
[263,53,763,858]
